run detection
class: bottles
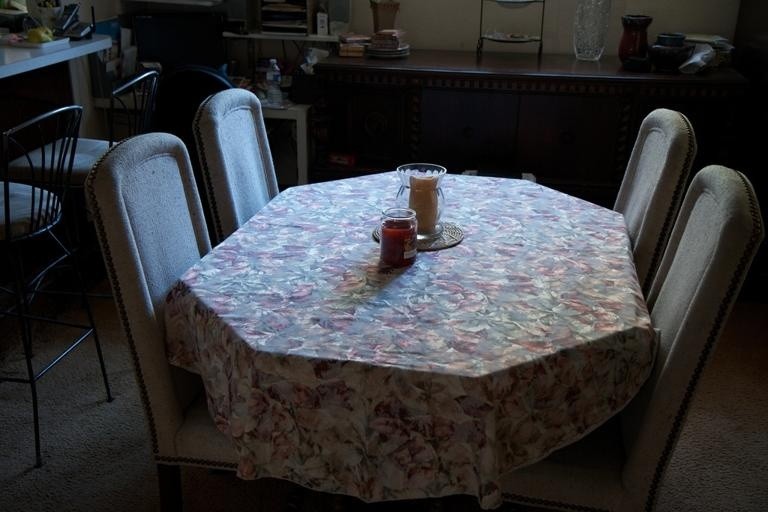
[619,15,653,67]
[265,59,283,107]
[396,163,445,239]
[647,32,696,68]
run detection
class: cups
[38,8,60,29]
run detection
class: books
[338,34,371,57]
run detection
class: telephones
[51,3,96,41]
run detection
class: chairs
[3,107,114,469]
[610,106,698,289]
[498,168,758,510]
[10,70,159,191]
[192,84,283,244]
[94,134,267,508]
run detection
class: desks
[1,32,113,139]
[177,158,653,511]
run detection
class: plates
[368,45,411,58]
